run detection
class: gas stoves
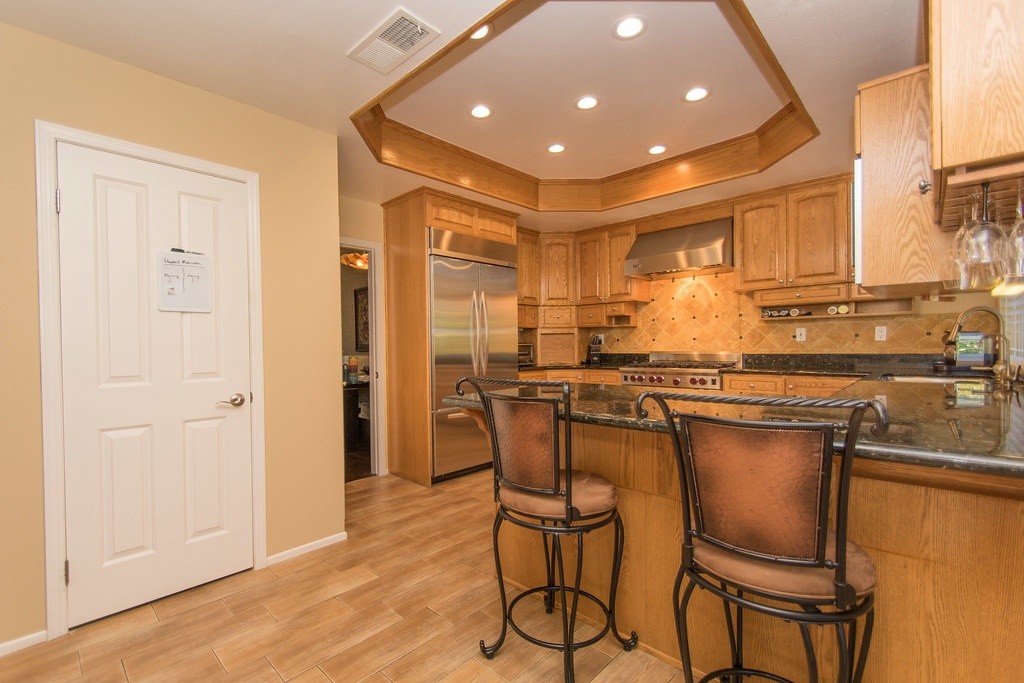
[618,359,736,390]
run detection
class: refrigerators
[429,255,518,481]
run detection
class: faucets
[945,304,1022,392]
[977,333,1012,400]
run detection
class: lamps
[340,249,369,269]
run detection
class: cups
[349,365,357,384]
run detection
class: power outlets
[875,327,886,342]
[796,328,805,341]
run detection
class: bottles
[343,364,348,384]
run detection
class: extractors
[623,217,734,281]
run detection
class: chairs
[455,376,638,682]
[634,388,890,683]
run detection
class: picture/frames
[354,286,369,353]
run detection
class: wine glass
[940,179,1024,291]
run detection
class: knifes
[590,334,602,345]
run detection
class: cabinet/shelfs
[343,387,360,453]
[734,0,1024,322]
[516,223,651,365]
